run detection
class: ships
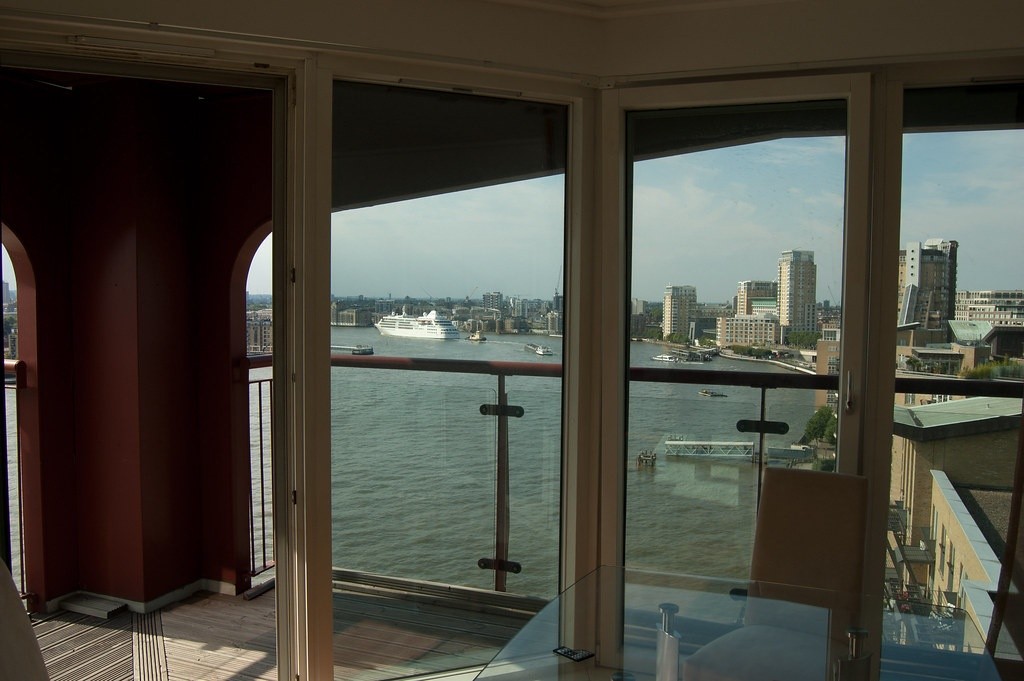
[374,305,461,339]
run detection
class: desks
[474,566,1003,681]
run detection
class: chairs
[683,466,873,681]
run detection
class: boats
[651,342,720,365]
[464,331,487,342]
[352,345,374,355]
[697,389,727,397]
[524,342,553,356]
